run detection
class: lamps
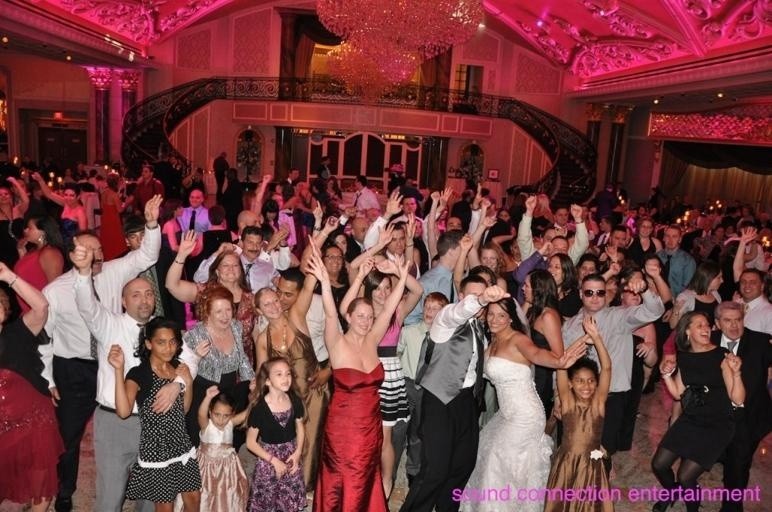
[86,66,140,92]
[313,0,482,109]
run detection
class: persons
[0,148,772,511]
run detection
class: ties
[145,268,166,315]
[470,319,484,404]
[727,342,737,350]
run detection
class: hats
[122,213,145,232]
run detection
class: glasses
[584,289,606,297]
